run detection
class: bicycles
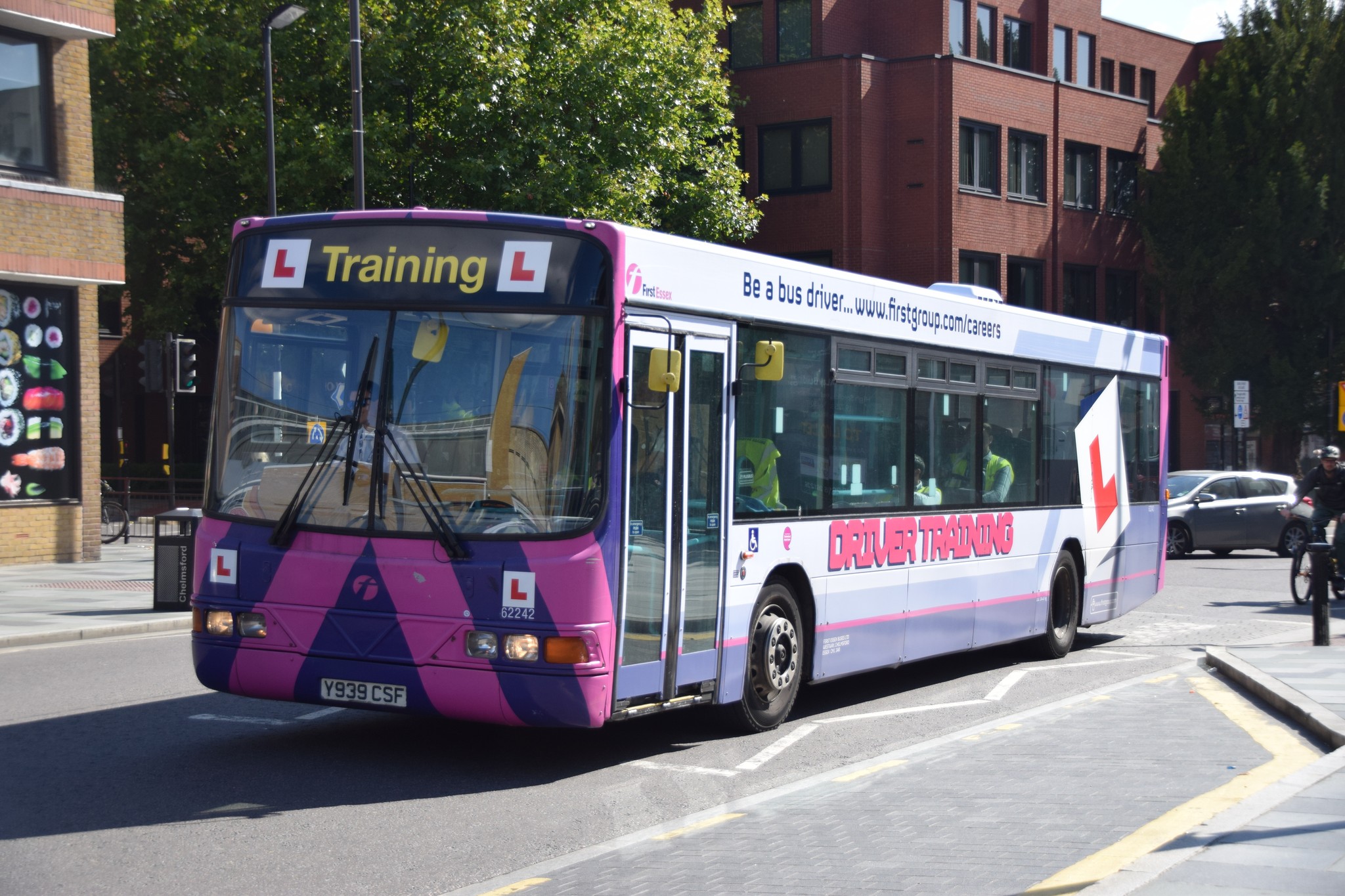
[100,481,129,544]
[1278,505,1345,605]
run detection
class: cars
[1166,470,1315,559]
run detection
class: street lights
[262,4,310,222]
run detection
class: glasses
[1322,459,1337,463]
[347,397,373,410]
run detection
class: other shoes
[1331,576,1345,591]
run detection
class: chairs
[1259,489,1275,496]
[1207,481,1229,501]
[1245,488,1259,497]
[1227,481,1241,499]
[940,414,1077,504]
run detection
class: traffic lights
[175,339,201,393]
[137,338,164,392]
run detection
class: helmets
[1320,446,1340,458]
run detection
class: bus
[191,206,1172,735]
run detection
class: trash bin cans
[154,509,203,612]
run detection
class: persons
[937,422,1015,504]
[874,452,942,507]
[1280,445,1345,592]
[336,380,422,483]
